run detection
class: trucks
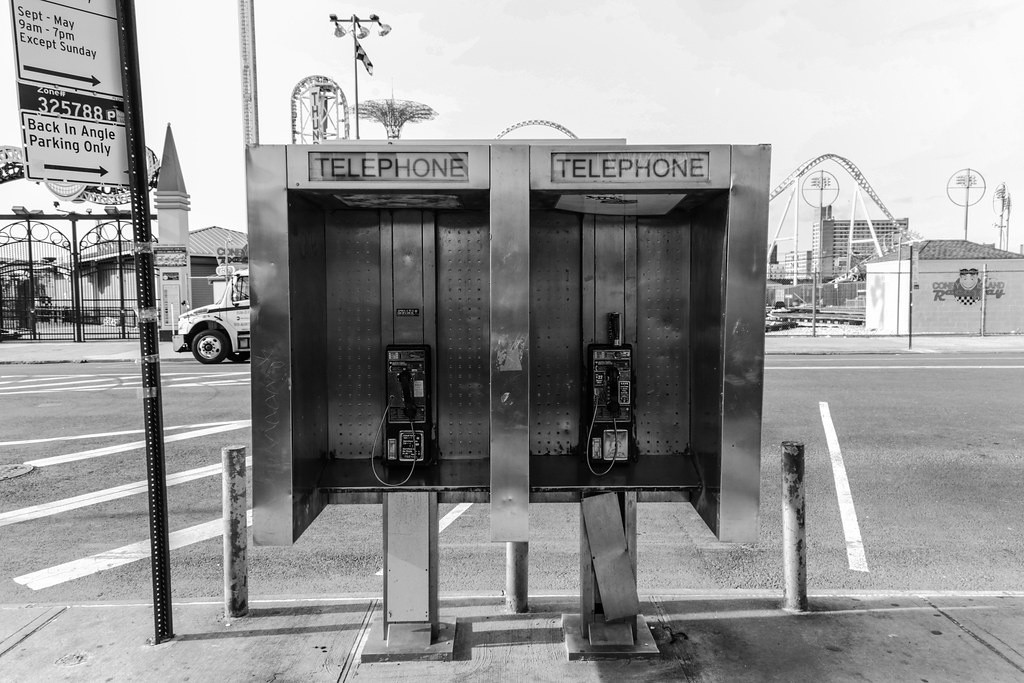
[171,266,251,364]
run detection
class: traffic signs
[8,0,124,104]
[18,107,133,186]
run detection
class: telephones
[384,342,431,425]
[587,342,636,425]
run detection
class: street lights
[103,205,132,339]
[11,205,44,340]
[327,13,391,140]
